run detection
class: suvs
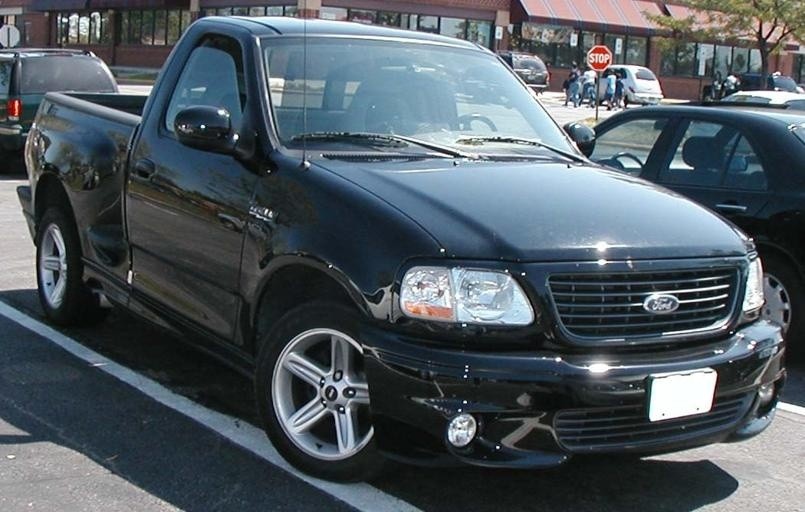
[457,48,550,90]
[703,71,800,104]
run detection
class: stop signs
[587,45,612,71]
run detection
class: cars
[578,62,664,106]
[0,45,119,174]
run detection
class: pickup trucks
[18,17,788,481]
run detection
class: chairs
[682,135,730,188]
[345,71,461,133]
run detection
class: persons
[562,60,628,111]
[713,72,743,95]
